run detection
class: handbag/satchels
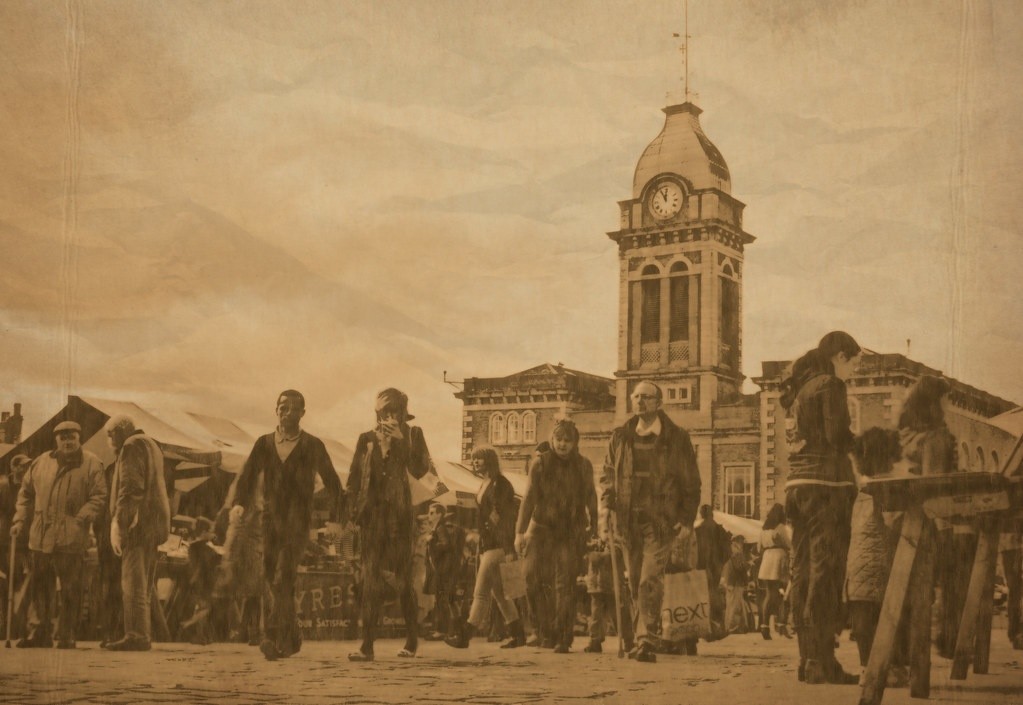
[404,425,449,504]
[659,539,712,639]
[499,553,532,600]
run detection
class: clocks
[648,180,684,220]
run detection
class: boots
[499,618,526,648]
[443,620,475,648]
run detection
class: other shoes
[397,647,416,657]
[347,649,374,660]
[106,637,152,651]
[15,633,55,648]
[584,642,602,653]
[760,626,771,638]
[799,658,860,686]
[57,637,76,648]
[777,624,789,638]
[633,643,655,660]
[529,631,571,653]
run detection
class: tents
[0,393,532,508]
[695,509,763,549]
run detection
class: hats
[10,454,33,467]
[53,421,81,435]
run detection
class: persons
[668,524,699,656]
[729,534,756,633]
[228,389,344,661]
[749,545,761,583]
[444,444,527,649]
[583,517,634,654]
[841,425,932,688]
[9,421,107,647]
[188,516,224,642]
[599,380,702,662]
[514,418,598,655]
[346,388,430,660]
[757,502,794,641]
[458,533,480,630]
[775,330,889,684]
[694,504,732,642]
[106,413,171,651]
[422,502,458,640]
[0,454,31,640]
[882,373,977,659]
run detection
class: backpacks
[715,523,733,559]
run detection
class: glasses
[632,393,660,401]
